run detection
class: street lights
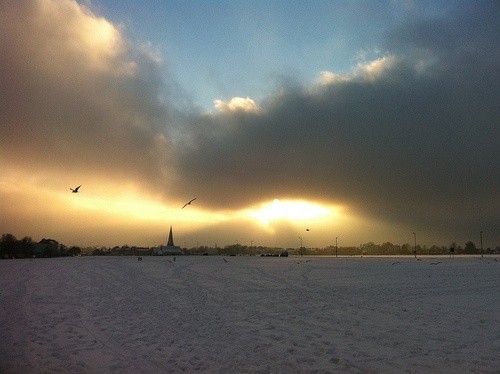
[412,231,416,258]
[298,236,302,256]
[335,236,337,256]
[479,231,483,258]
[251,240,253,255]
[235,240,238,256]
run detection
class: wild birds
[70,185,81,193]
[181,197,197,210]
